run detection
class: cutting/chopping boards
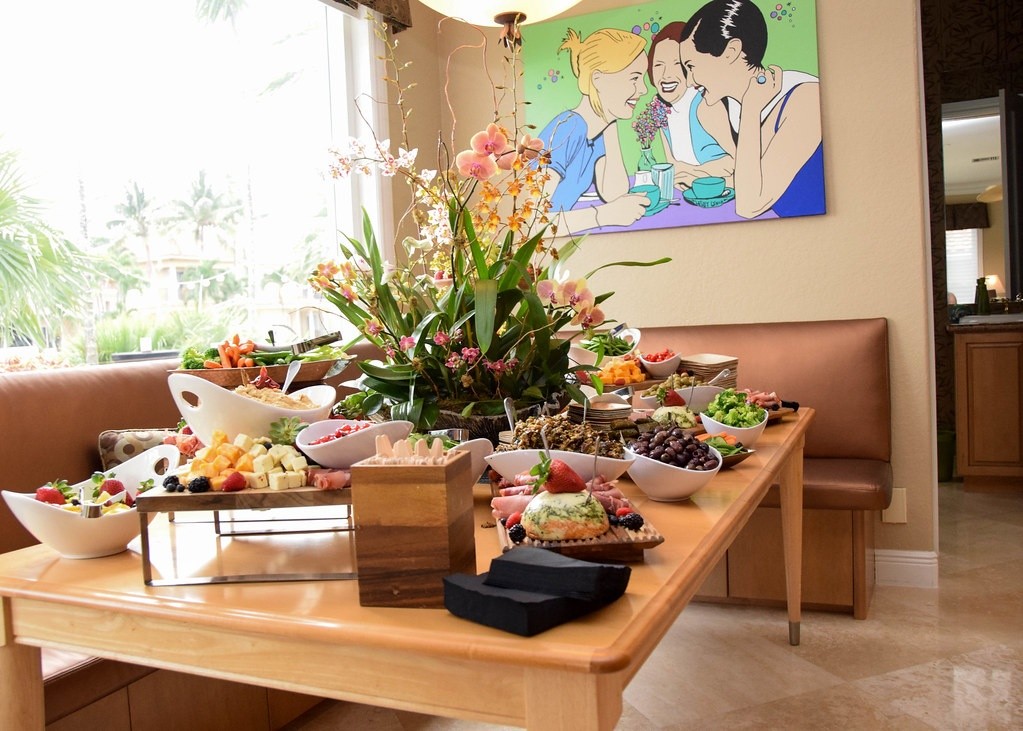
[766,408,794,420]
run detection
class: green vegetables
[405,431,464,451]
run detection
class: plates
[643,198,670,216]
[136,464,352,512]
[682,187,735,208]
[489,478,665,554]
[677,354,738,389]
[567,403,632,432]
[166,354,358,386]
[721,449,756,469]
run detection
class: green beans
[577,331,635,356]
[704,437,746,456]
[244,350,296,365]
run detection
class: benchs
[0,318,895,731]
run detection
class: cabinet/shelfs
[955,330,1023,492]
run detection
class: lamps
[417,0,579,53]
[984,274,1007,299]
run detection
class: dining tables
[0,406,817,731]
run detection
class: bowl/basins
[447,438,494,488]
[484,446,637,487]
[567,328,641,370]
[640,353,682,379]
[296,419,414,469]
[640,386,725,415]
[700,410,769,448]
[1,444,180,559]
[168,373,336,447]
[568,385,629,405]
[623,446,722,502]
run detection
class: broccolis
[179,345,221,369]
[702,388,765,428]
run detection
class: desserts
[594,356,646,384]
[176,430,311,489]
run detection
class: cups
[692,177,726,199]
[628,185,661,211]
[651,163,674,200]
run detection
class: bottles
[634,171,655,186]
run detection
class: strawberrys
[656,388,687,406]
[176,417,193,435]
[527,451,588,495]
[91,471,134,506]
[136,478,156,496]
[35,477,76,504]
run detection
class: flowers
[302,15,673,432]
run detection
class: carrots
[695,432,736,444]
[203,332,256,368]
